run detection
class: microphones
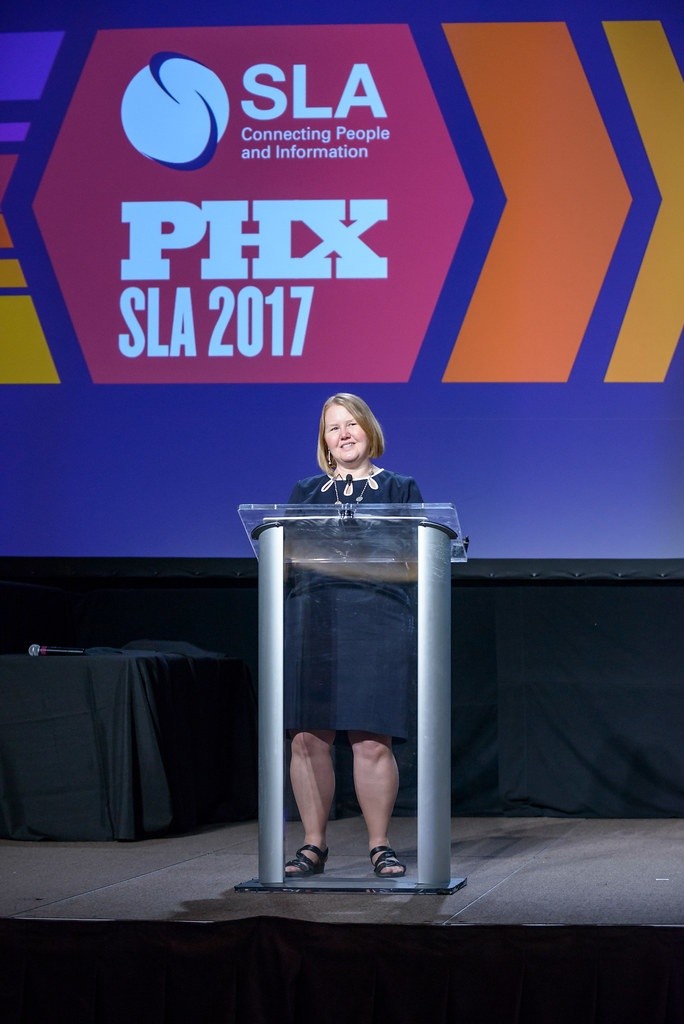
[29,644,84,657]
[347,474,353,486]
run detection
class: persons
[282,392,427,878]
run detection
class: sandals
[370,846,406,877]
[285,844,329,877]
